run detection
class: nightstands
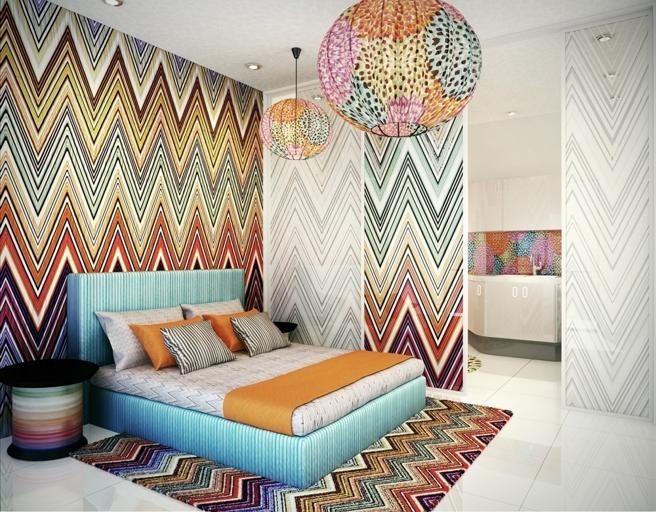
[0,359,99,460]
[273,322,297,342]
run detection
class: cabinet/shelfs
[468,280,561,343]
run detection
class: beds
[66,269,426,489]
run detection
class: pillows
[181,298,244,321]
[160,320,236,375]
[203,308,260,352]
[230,312,291,357]
[128,316,203,370]
[95,306,184,372]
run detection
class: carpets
[70,395,514,512]
[467,355,481,372]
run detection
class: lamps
[259,48,332,159]
[317,0,482,137]
